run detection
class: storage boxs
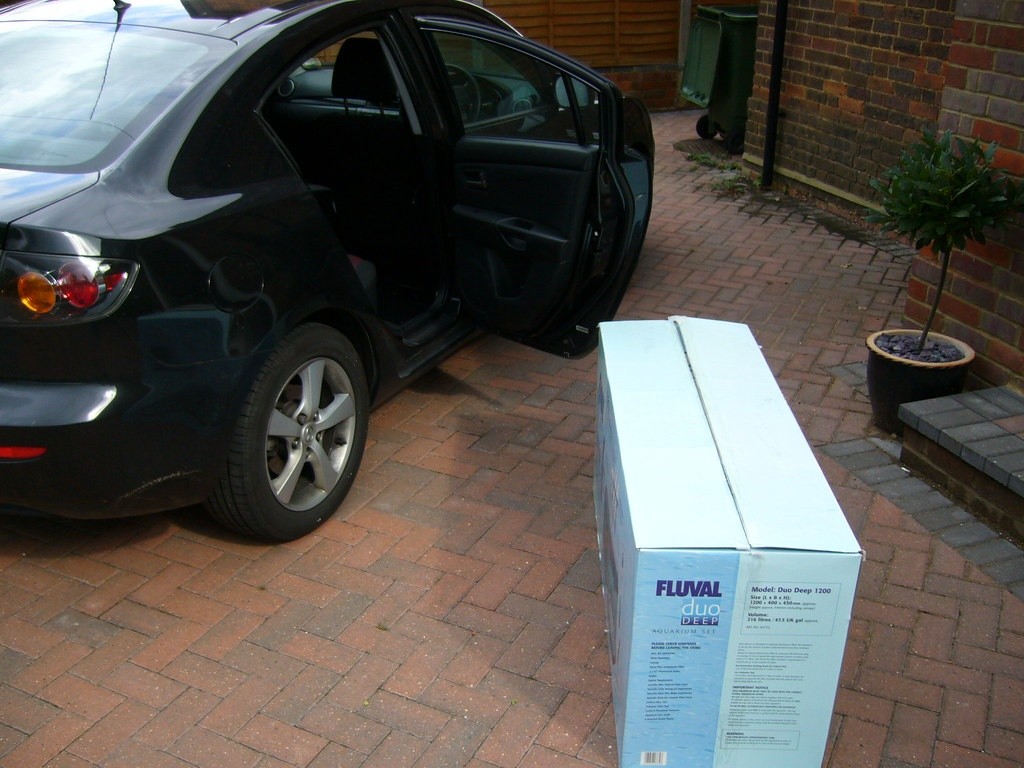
[590,316,862,768]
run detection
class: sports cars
[0,0,657,544]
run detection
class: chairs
[330,38,395,106]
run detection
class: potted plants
[863,131,1014,435]
